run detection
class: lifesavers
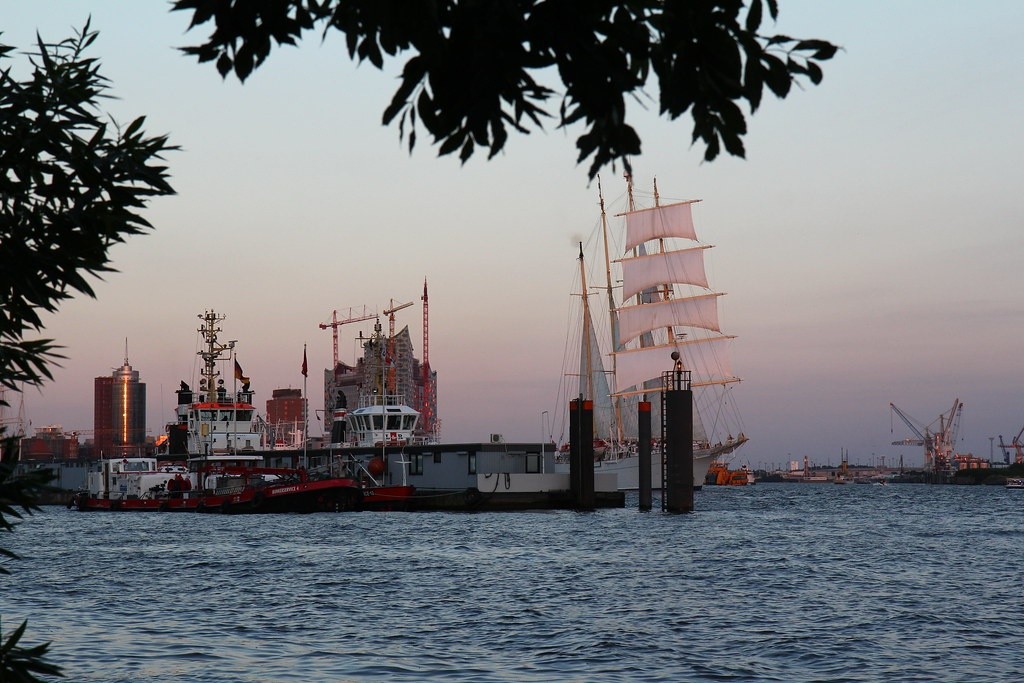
[196,501,203,512]
[220,498,230,513]
[250,491,264,508]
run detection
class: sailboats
[554,174,751,487]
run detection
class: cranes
[382,297,413,354]
[889,390,963,462]
[319,304,380,369]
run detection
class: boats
[70,489,123,510]
[360,476,416,515]
[199,466,254,514]
[164,487,203,512]
[251,461,358,512]
[835,476,855,485]
[119,493,166,511]
